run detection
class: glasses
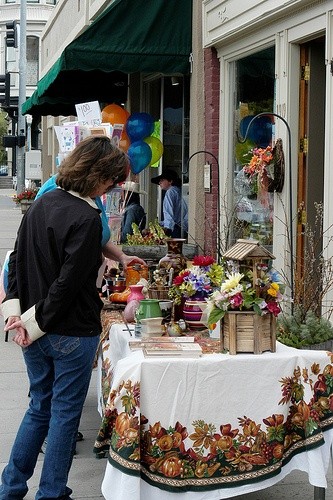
[111,175,119,181]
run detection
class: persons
[120,181,146,244]
[0,136,127,500]
[151,171,188,238]
[0,174,147,307]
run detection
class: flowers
[241,146,273,186]
[201,263,287,331]
[168,255,227,306]
[12,187,40,205]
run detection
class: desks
[93,293,333,500]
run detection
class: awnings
[21,0,76,116]
[37,1,192,96]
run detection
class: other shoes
[76,432,83,441]
[40,437,49,455]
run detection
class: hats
[121,181,147,194]
[151,169,189,186]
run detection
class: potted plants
[122,219,168,262]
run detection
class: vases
[183,296,209,331]
[126,285,163,324]
[20,198,33,214]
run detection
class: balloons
[102,105,163,175]
[234,115,275,165]
